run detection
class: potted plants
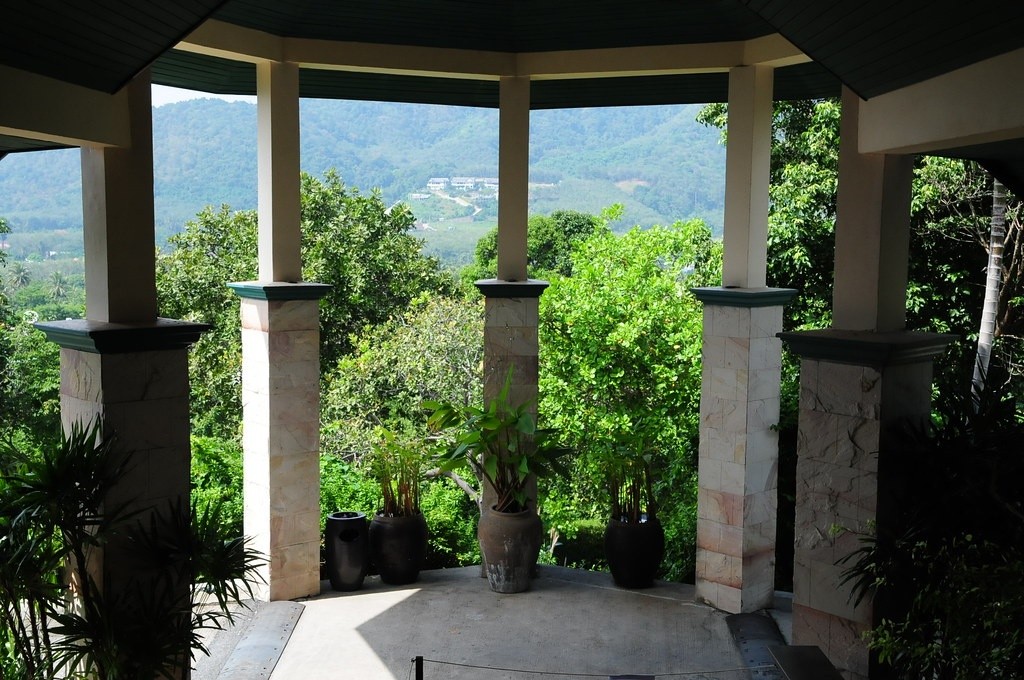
[419,364,576,593]
[369,426,429,585]
[599,437,665,590]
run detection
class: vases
[324,510,369,592]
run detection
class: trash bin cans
[326,510,369,592]
